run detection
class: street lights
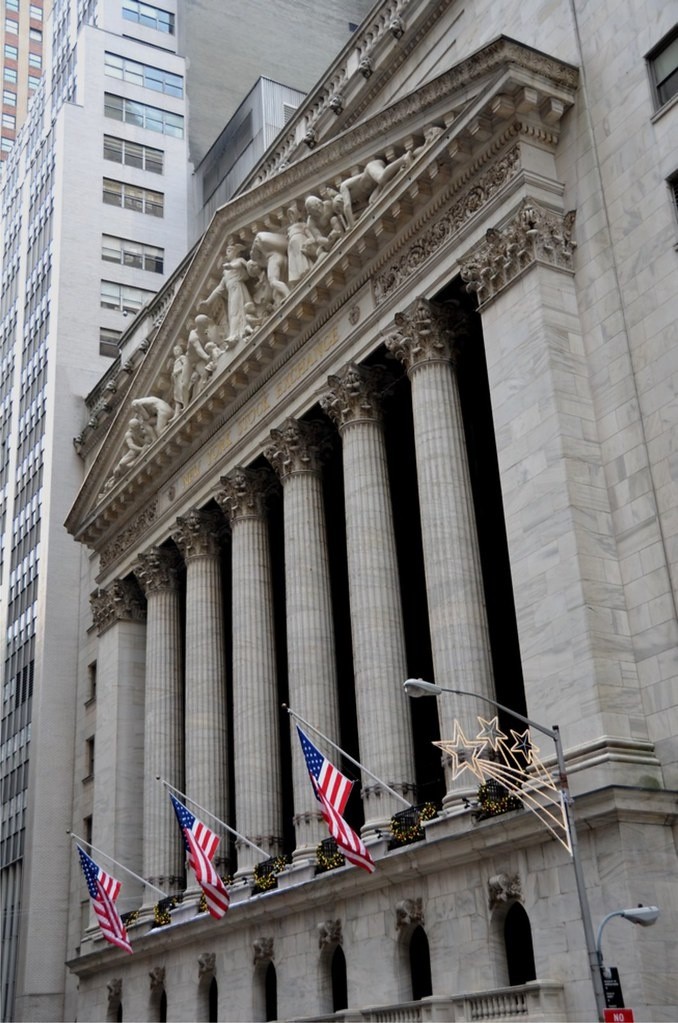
[403,678,660,1023]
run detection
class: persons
[114,138,430,477]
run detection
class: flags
[170,792,230,919]
[76,843,134,955]
[296,725,376,873]
